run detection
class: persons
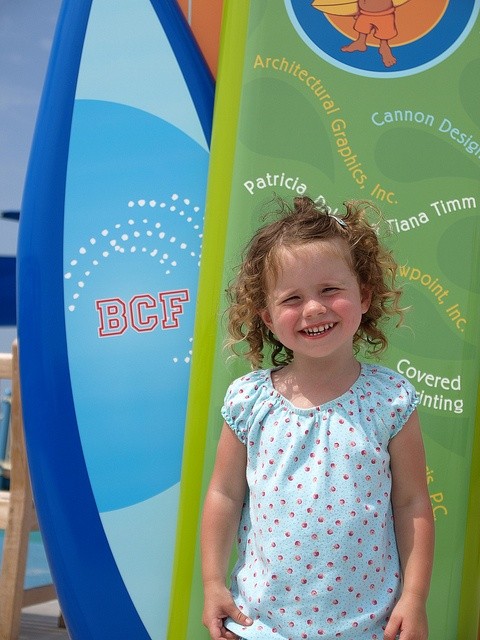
[200,195,435,640]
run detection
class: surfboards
[16,0,215,639]
[165,0,480,640]
